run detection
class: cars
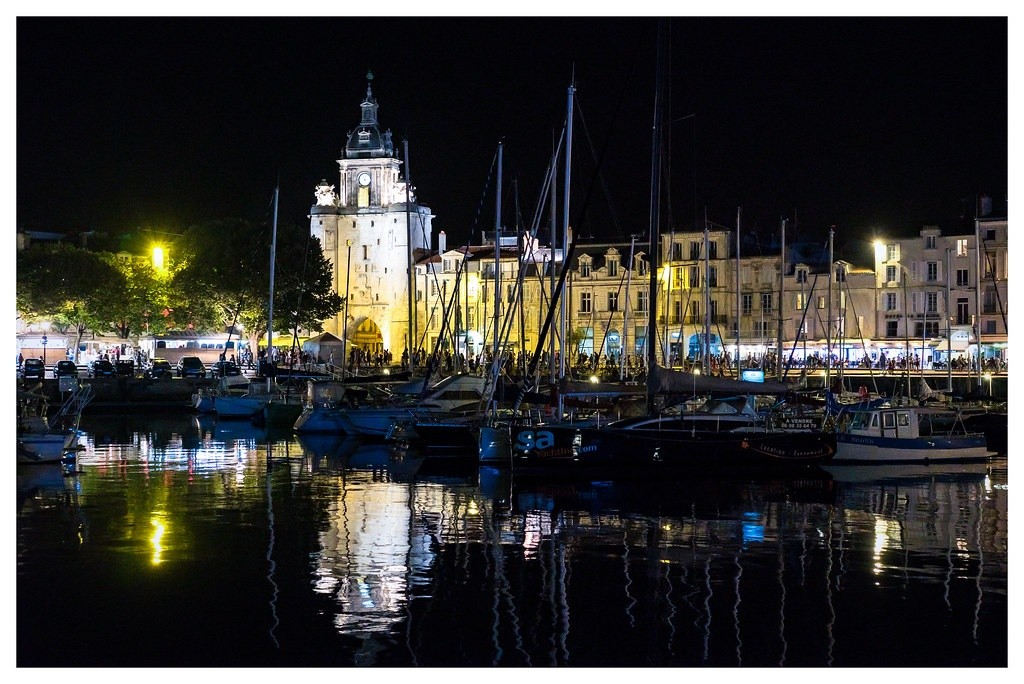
[86,359,119,379]
[176,355,207,379]
[211,360,240,378]
[53,360,78,378]
[19,358,46,380]
[144,359,172,378]
[254,356,279,377]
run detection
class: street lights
[867,234,889,341]
[41,336,47,366]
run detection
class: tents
[303,332,343,368]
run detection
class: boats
[16,382,96,463]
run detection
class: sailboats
[190,62,1007,480]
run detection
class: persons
[17,353,24,370]
[843,414,852,434]
[66,345,147,370]
[219,343,1008,375]
[39,356,44,364]
